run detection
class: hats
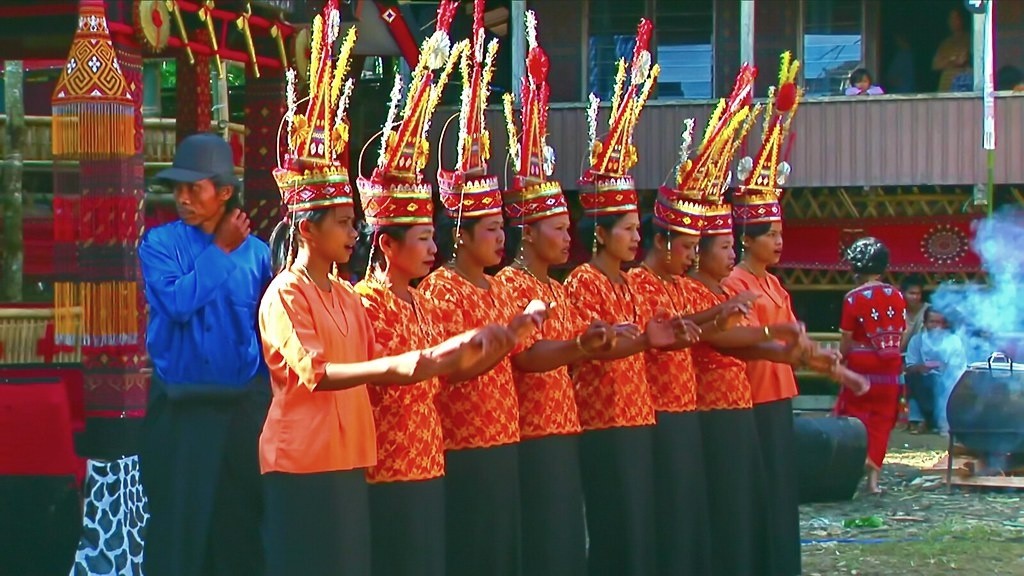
[155,132,233,186]
[271,0,369,211]
[435,0,505,216]
[500,9,569,227]
[578,17,660,216]
[653,51,804,237]
[356,0,468,226]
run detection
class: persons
[997,66,1024,93]
[259,165,871,576]
[883,32,918,92]
[845,69,886,96]
[833,236,907,493]
[931,9,970,92]
[138,132,274,576]
[900,276,969,437]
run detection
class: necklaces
[296,258,349,337]
[642,261,686,315]
[590,259,637,323]
[514,258,566,322]
[369,271,432,341]
[687,272,729,303]
[741,260,784,308]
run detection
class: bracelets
[577,333,590,358]
[763,325,772,340]
[714,317,726,332]
